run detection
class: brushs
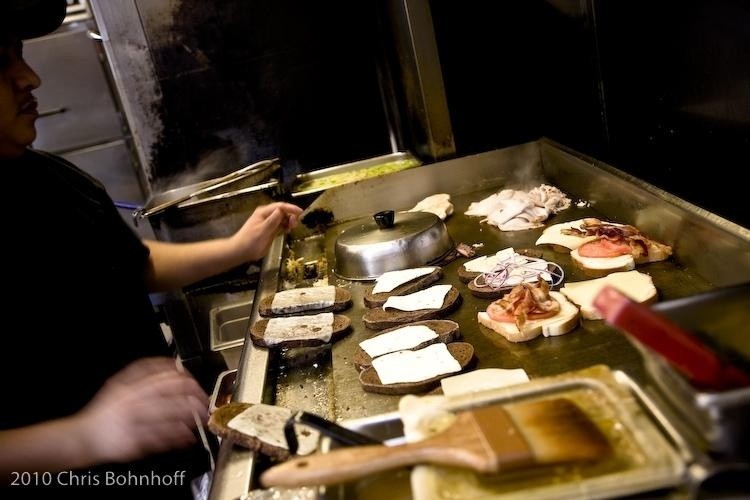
[261,398,613,488]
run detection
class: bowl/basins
[693,464,750,499]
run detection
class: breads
[207,401,323,458]
[248,218,673,397]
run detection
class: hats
[0,0,66,37]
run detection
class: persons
[2,0,305,499]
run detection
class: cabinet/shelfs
[21,1,165,311]
